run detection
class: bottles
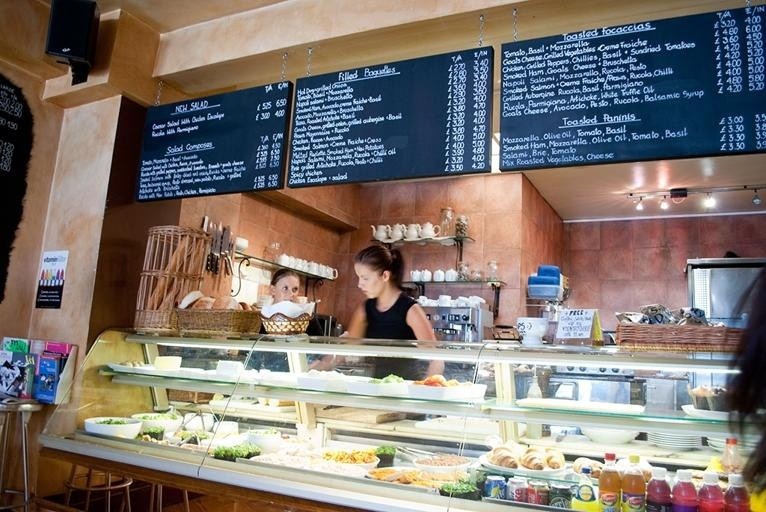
[599,452,621,512]
[454,215,472,236]
[455,262,470,282]
[698,470,724,512]
[646,467,672,512]
[439,207,455,238]
[720,438,744,472]
[621,455,646,512]
[524,375,543,438]
[724,474,751,512]
[485,259,503,281]
[671,469,698,512]
[572,467,600,511]
[471,267,484,282]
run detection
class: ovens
[548,375,631,404]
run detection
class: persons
[237,269,336,373]
[313,246,444,385]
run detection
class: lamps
[704,192,713,206]
[659,196,669,211]
[632,196,644,211]
[751,189,760,208]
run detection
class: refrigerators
[683,258,766,404]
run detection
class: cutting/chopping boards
[209,398,296,415]
[316,406,405,423]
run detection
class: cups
[409,269,420,282]
[446,268,457,281]
[421,268,432,282]
[433,269,444,281]
[277,253,338,280]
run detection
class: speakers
[38,0,105,69]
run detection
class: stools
[0,401,43,512]
[64,463,134,512]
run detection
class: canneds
[486,476,572,508]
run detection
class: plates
[367,467,421,478]
[681,405,728,420]
[707,438,761,456]
[648,431,702,452]
[479,455,573,478]
[412,456,473,470]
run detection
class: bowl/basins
[84,416,142,439]
[685,385,725,410]
[152,355,182,369]
[355,456,380,469]
[514,316,557,346]
[214,419,237,438]
[581,427,640,445]
[131,413,183,435]
[185,413,213,432]
[213,360,247,373]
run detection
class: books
[0,336,71,405]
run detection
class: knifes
[556,429,568,444]
[207,225,233,277]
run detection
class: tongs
[396,444,443,461]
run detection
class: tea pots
[402,223,423,239]
[371,224,392,240]
[387,223,408,239]
[416,222,441,237]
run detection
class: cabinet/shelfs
[38,326,760,512]
[371,236,507,320]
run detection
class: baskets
[166,389,215,403]
[256,313,314,335]
[179,309,260,339]
[616,323,746,350]
